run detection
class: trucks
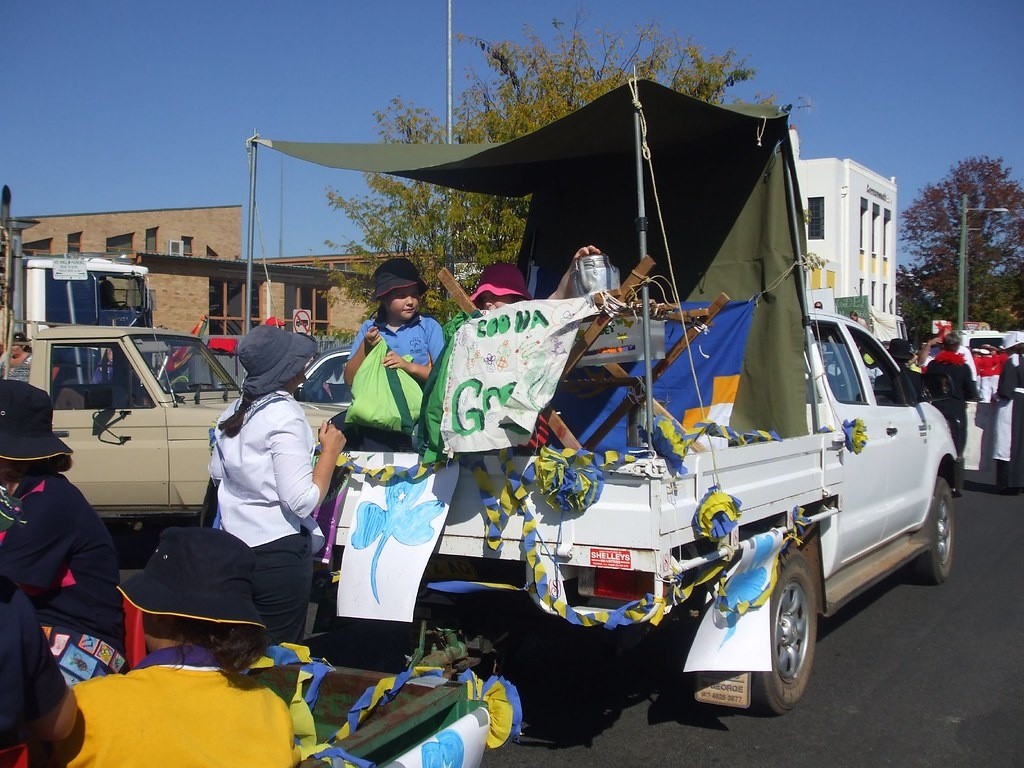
[332,307,965,718]
[1,184,153,385]
[0,310,348,518]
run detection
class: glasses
[815,306,821,308]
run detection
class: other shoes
[999,486,1020,495]
[952,491,962,497]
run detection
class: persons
[849,311,868,330]
[0,329,119,768]
[469,245,602,449]
[326,256,444,455]
[813,302,822,309]
[28,526,301,768]
[858,332,1024,496]
[266,317,287,328]
[210,326,347,647]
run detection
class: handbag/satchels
[345,335,422,435]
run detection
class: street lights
[958,194,1009,329]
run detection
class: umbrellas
[166,314,209,371]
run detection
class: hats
[0,486,27,532]
[117,527,266,628]
[470,264,533,301]
[238,326,317,396]
[0,380,73,460]
[999,331,1024,349]
[981,344,991,349]
[14,333,28,341]
[972,348,979,352]
[979,349,990,354]
[989,346,999,351]
[888,338,914,359]
[373,258,428,300]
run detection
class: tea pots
[570,254,620,298]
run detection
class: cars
[291,344,354,407]
